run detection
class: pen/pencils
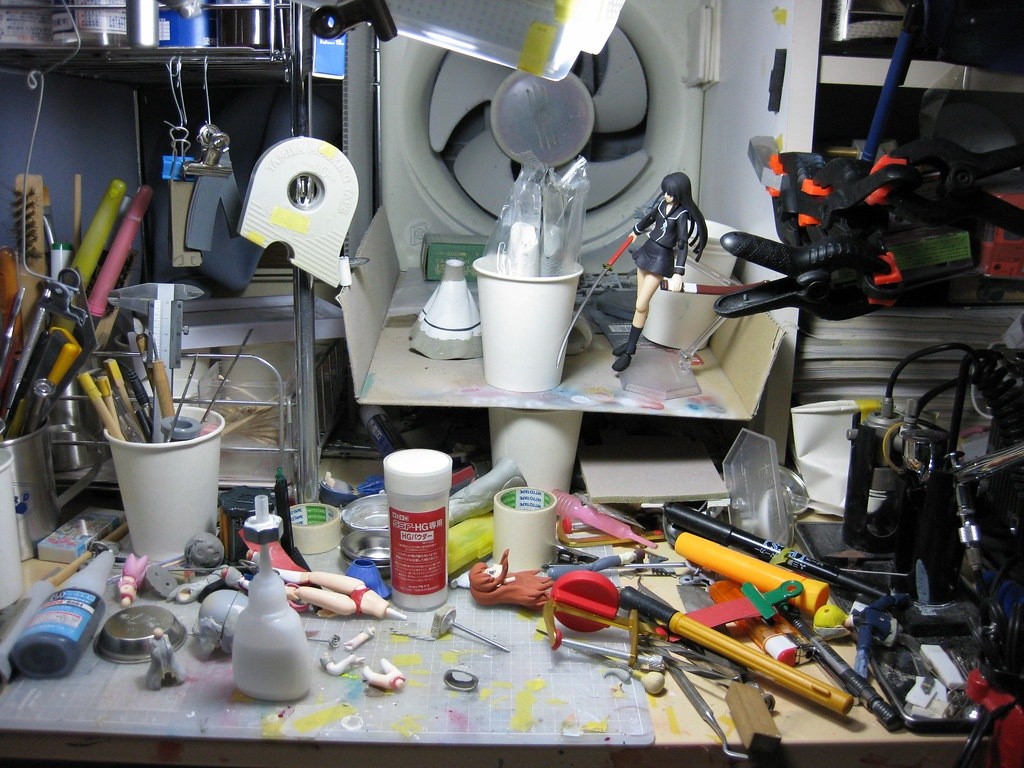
[795,617,901,732]
[664,501,892,598]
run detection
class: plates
[340,493,391,579]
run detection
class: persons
[470,549,554,611]
[318,626,406,692]
[612,171,708,371]
[811,591,916,680]
[219,549,390,616]
[547,547,646,582]
[145,627,189,693]
[117,550,152,608]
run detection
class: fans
[379,0,704,272]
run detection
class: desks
[0,456,1023,762]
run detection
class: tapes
[289,504,343,554]
[493,487,558,573]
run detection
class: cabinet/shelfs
[699,0,1024,467]
[0,1,348,514]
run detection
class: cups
[489,407,582,494]
[104,407,226,563]
[0,448,31,610]
[0,416,59,542]
[791,401,897,517]
[643,218,742,350]
[472,257,585,393]
